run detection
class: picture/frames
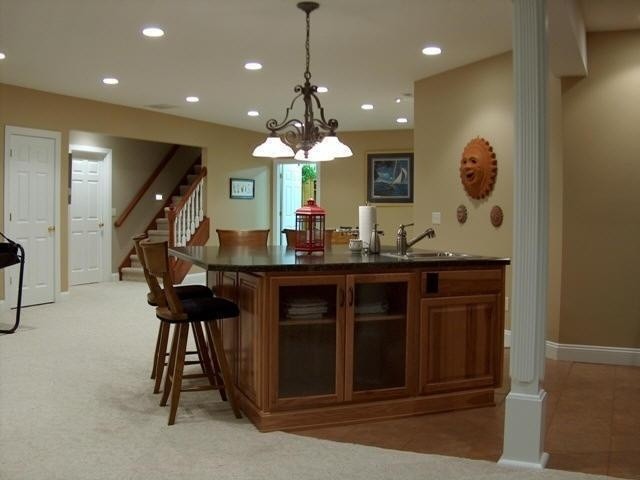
[363,149,414,208]
[228,177,256,200]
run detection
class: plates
[283,300,328,320]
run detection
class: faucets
[397,223,436,254]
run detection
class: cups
[348,238,363,253]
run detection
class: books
[285,298,329,320]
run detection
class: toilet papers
[358,206,377,249]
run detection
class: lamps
[248,0,354,164]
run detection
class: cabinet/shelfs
[199,263,419,432]
[416,257,510,415]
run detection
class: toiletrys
[370,223,380,254]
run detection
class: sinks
[411,253,460,258]
